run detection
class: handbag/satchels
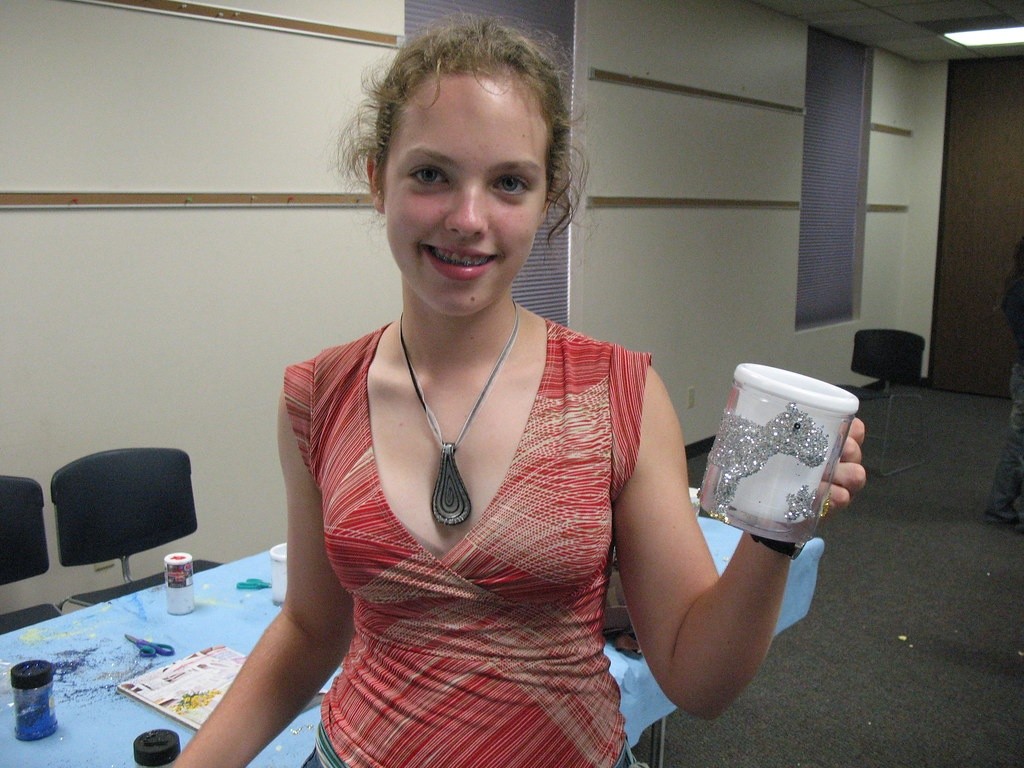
[602,543,642,655]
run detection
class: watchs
[750,534,807,560]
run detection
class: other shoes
[984,507,1024,522]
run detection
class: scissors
[237,578,272,589]
[125,633,175,657]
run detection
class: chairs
[836,330,926,479]
[0,447,225,640]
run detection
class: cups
[269,541,288,607]
[697,362,860,545]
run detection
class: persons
[172,18,867,768]
[982,235,1024,525]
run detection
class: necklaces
[400,298,520,525]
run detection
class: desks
[0,488,824,766]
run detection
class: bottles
[10,661,58,742]
[164,552,197,614]
[133,728,181,768]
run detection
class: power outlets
[688,386,695,408]
